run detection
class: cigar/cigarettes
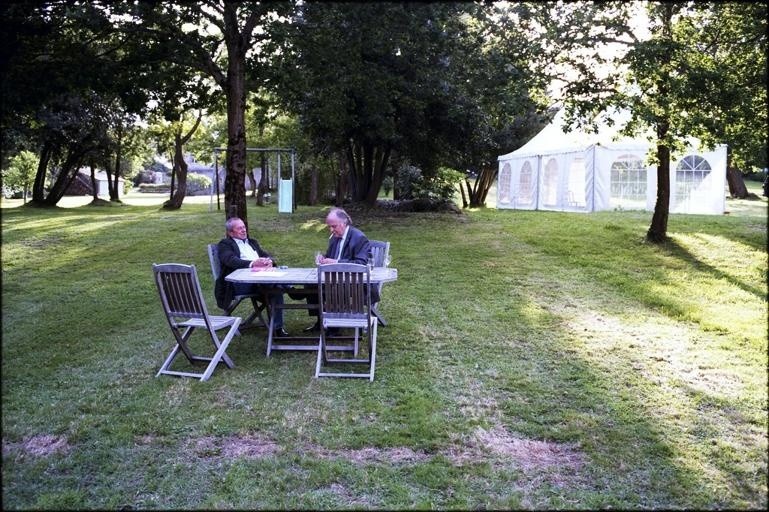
[329,233,334,240]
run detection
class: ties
[334,238,342,259]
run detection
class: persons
[304,206,374,334]
[215,217,309,337]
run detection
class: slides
[277,178,293,213]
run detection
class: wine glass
[383,255,392,271]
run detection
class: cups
[315,251,322,264]
[368,258,375,272]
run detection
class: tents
[497,104,728,214]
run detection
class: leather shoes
[275,328,289,336]
[289,289,307,300]
[302,323,321,332]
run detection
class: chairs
[321,237,393,338]
[144,259,246,384]
[206,242,287,340]
[310,261,380,384]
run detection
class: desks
[219,265,402,361]
